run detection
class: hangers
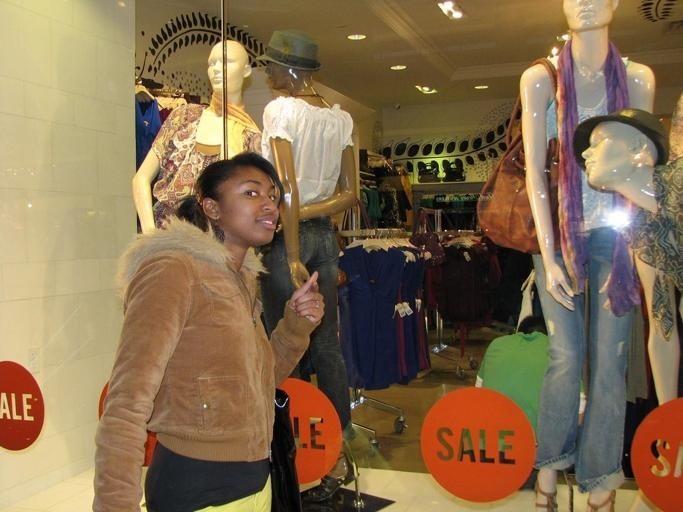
[344,228,430,263]
[444,230,487,252]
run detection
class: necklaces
[290,90,321,101]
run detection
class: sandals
[587,490,615,512]
[534,481,557,512]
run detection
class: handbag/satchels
[271,386,302,511]
[476,58,561,254]
[409,208,447,268]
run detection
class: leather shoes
[306,459,359,503]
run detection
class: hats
[255,29,320,70]
[571,107,669,170]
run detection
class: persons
[132,38,261,234]
[253,29,357,502]
[520,1,656,510]
[570,106,681,512]
[90,152,326,512]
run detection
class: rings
[313,299,320,309]
